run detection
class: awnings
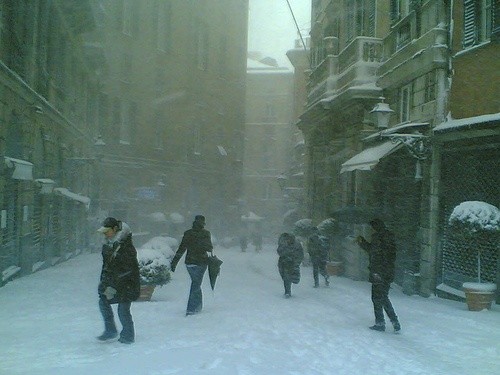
[339,138,413,174]
[54,187,91,208]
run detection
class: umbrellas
[207,250,223,292]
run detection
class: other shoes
[393,323,399,331]
[370,325,385,331]
[285,294,290,297]
[98,333,117,341]
[186,311,194,316]
[117,337,130,344]
[326,276,330,286]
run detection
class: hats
[97,218,117,233]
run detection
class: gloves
[104,285,116,300]
[171,264,176,272]
[357,235,364,241]
[369,276,376,282]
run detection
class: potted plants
[448,201,500,311]
[293,218,343,276]
[138,258,171,301]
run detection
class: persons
[95,217,141,343]
[306,225,331,290]
[239,235,263,253]
[169,215,213,315]
[356,217,401,334]
[276,232,304,298]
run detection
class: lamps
[368,94,432,161]
